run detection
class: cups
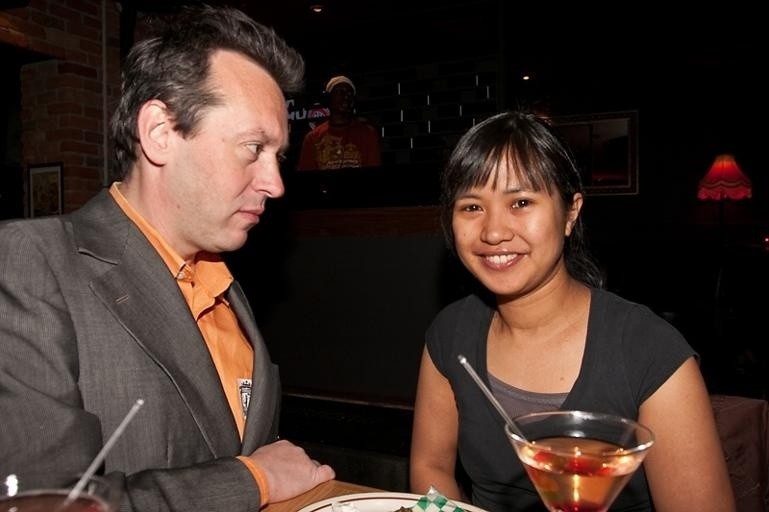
[2,489,112,511]
[503,410,656,511]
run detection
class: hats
[326,75,356,95]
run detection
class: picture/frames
[27,162,63,217]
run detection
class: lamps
[699,153,751,224]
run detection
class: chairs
[711,394,768,512]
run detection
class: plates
[286,491,485,512]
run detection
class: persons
[300,74,383,171]
[1,0,337,511]
[410,113,736,511]
